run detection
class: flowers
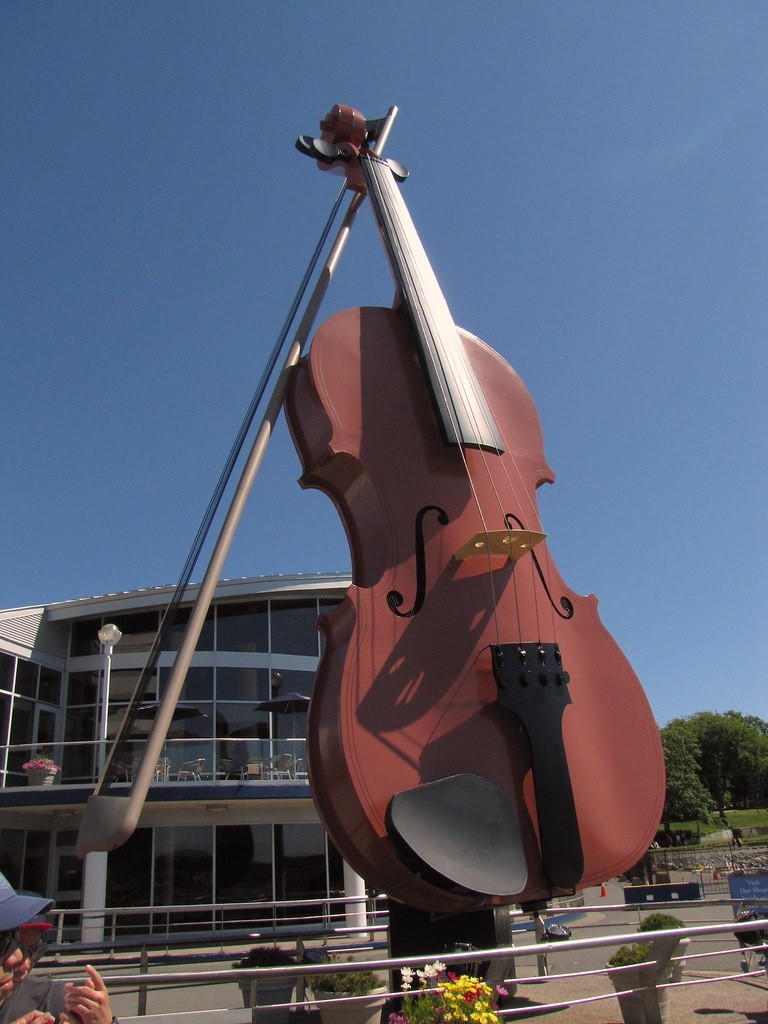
[387,960,509,1024]
[22,751,61,770]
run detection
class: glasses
[0,922,22,958]
[21,944,46,963]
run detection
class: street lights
[99,624,123,782]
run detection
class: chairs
[98,753,304,785]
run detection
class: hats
[0,871,55,932]
[13,889,54,929]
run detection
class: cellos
[275,101,670,919]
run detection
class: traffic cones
[713,871,718,879]
[600,883,608,897]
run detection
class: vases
[25,768,57,786]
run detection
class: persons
[1,872,120,1024]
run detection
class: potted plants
[305,952,389,1024]
[605,942,671,1024]
[232,946,320,1024]
[638,912,690,984]
